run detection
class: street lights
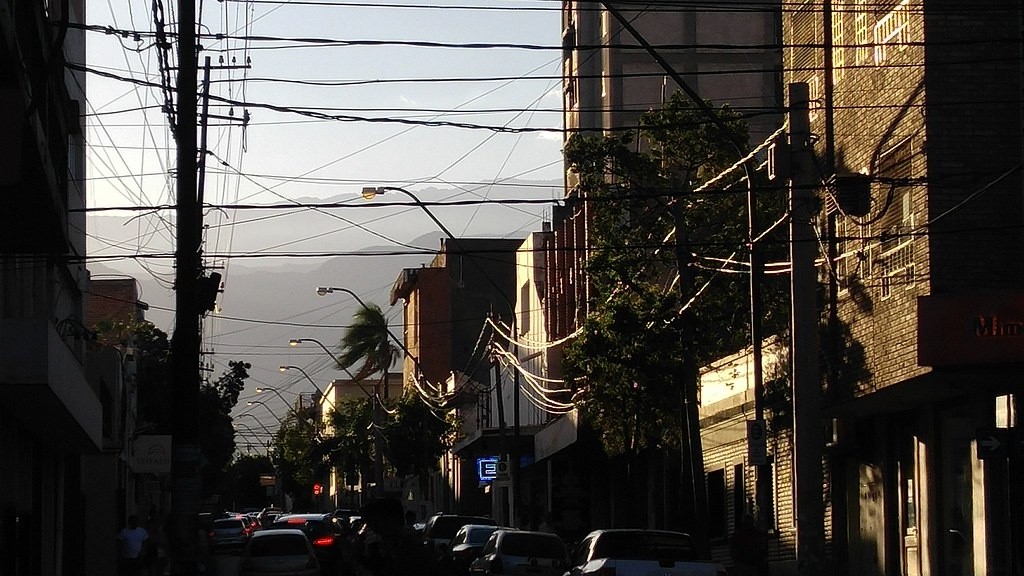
[318,287,450,514]
[289,339,382,498]
[363,186,521,528]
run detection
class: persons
[118,515,149,576]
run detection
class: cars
[193,502,730,576]
